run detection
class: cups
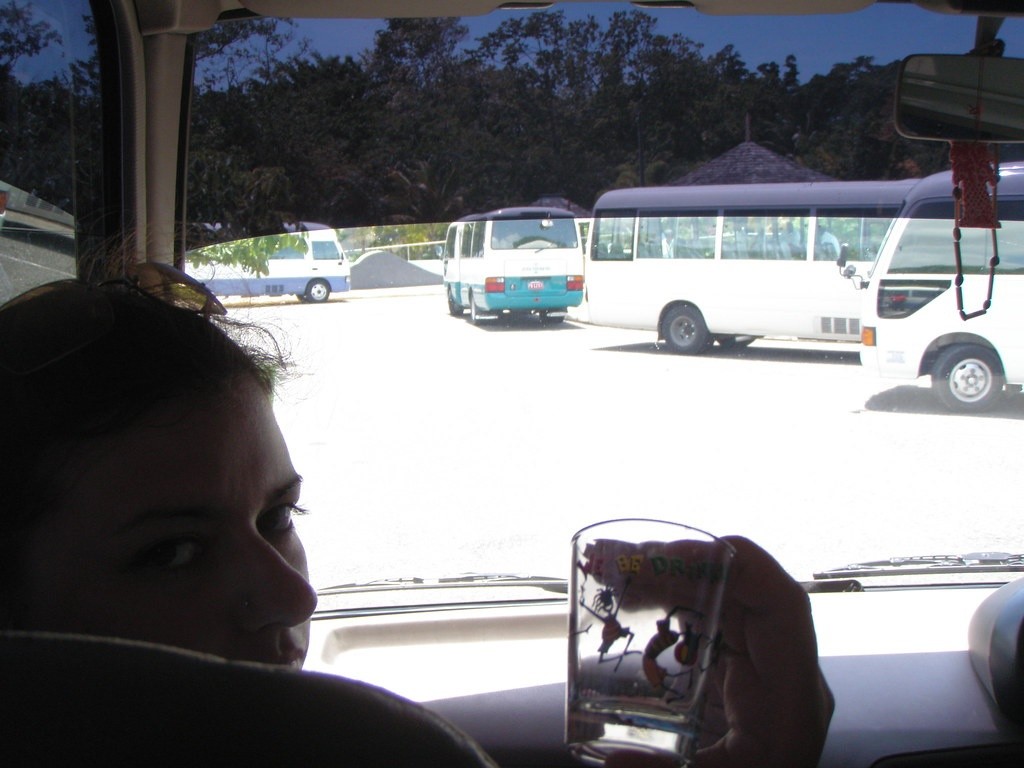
[568,519,736,764]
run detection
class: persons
[0,240,836,768]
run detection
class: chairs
[598,236,839,261]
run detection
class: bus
[577,176,922,356]
[178,220,354,305]
[859,156,1024,414]
[441,205,587,327]
